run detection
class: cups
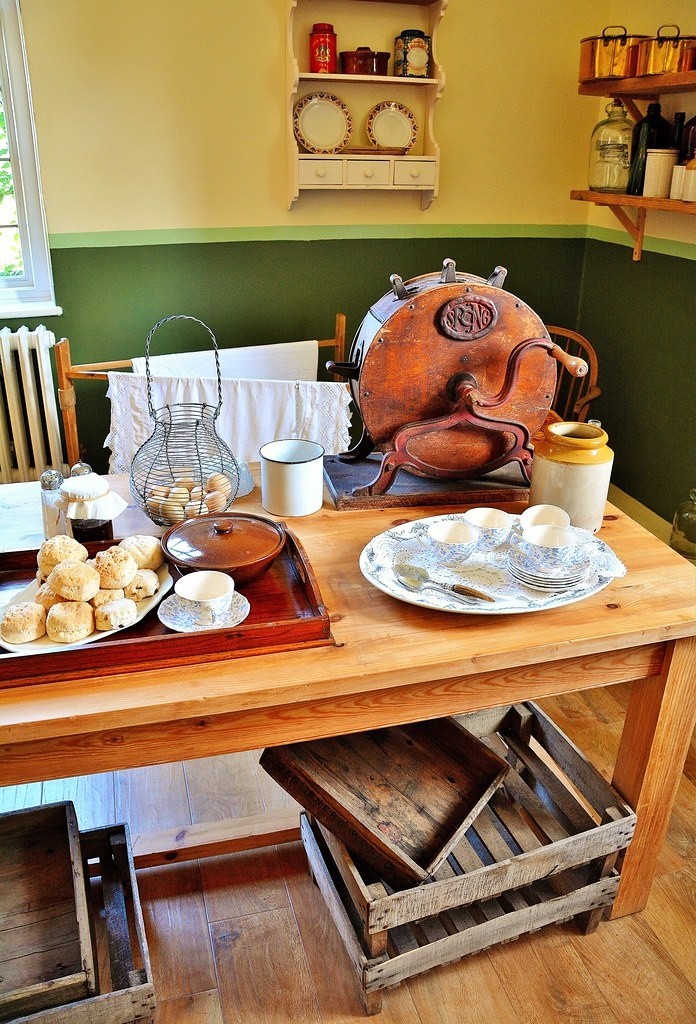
[258,439,325,517]
[174,571,235,626]
[417,503,574,574]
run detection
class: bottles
[40,460,114,543]
[588,107,635,195]
[631,103,696,196]
[528,420,614,530]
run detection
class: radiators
[0,323,69,484]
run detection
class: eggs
[143,473,231,525]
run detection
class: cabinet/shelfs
[569,70,695,260]
[288,1,448,210]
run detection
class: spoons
[392,565,495,602]
[397,575,480,604]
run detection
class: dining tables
[0,460,696,919]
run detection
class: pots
[340,47,391,76]
[636,23,696,76]
[578,25,653,84]
[160,512,287,586]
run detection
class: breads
[0,534,164,643]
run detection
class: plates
[360,513,617,615]
[157,591,252,633]
[293,92,354,155]
[0,563,174,653]
[366,101,419,154]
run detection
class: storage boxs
[0,801,157,1024]
[260,700,638,1015]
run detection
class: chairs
[544,326,602,422]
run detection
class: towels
[129,339,319,382]
